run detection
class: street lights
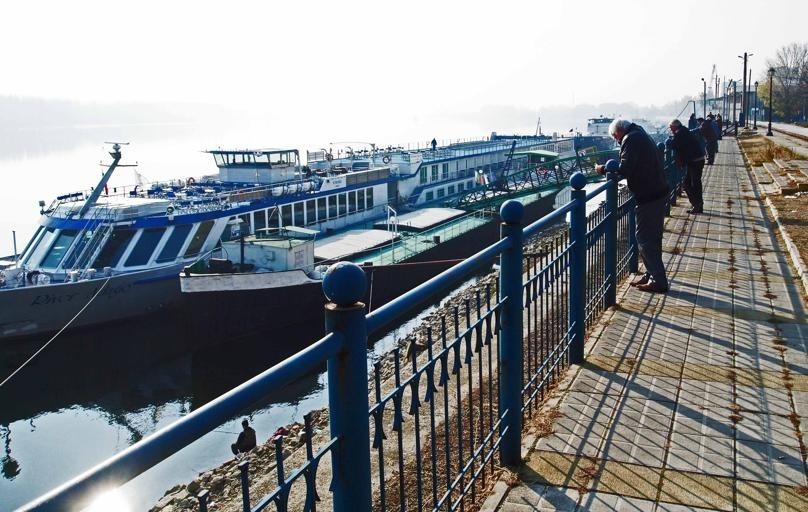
[728,52,775,136]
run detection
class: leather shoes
[629,278,647,286]
[638,283,659,292]
[686,209,703,214]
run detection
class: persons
[430,138,437,151]
[670,119,708,215]
[688,111,724,166]
[588,115,671,294]
